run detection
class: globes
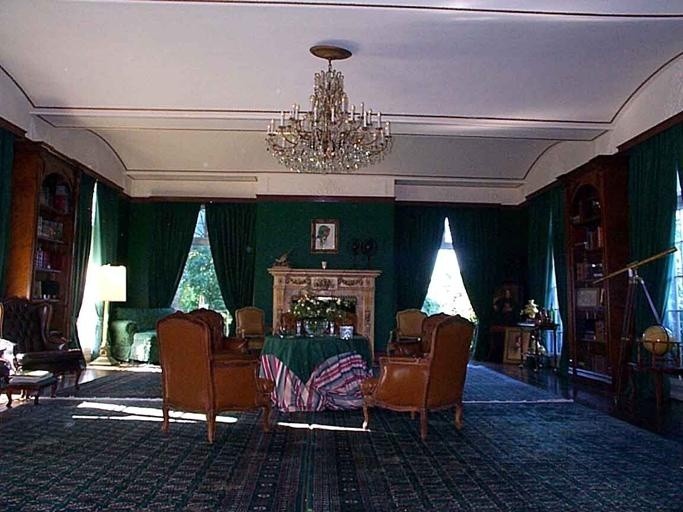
[609,325,683,423]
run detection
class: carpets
[0,361,681,509]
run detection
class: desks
[518,324,558,372]
[620,339,682,415]
[258,334,373,414]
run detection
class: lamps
[265,44,394,175]
[93,264,127,365]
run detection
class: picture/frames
[501,326,520,364]
[575,287,598,308]
[307,215,339,256]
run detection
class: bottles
[520,299,557,326]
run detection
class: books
[568,194,608,376]
[34,182,69,301]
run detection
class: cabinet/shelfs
[5,142,82,345]
[555,154,628,407]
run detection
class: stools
[5,375,57,409]
[132,331,159,363]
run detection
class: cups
[340,325,354,340]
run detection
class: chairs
[280,309,357,336]
[154,306,272,442]
[358,308,475,442]
[113,306,176,363]
[0,297,84,398]
